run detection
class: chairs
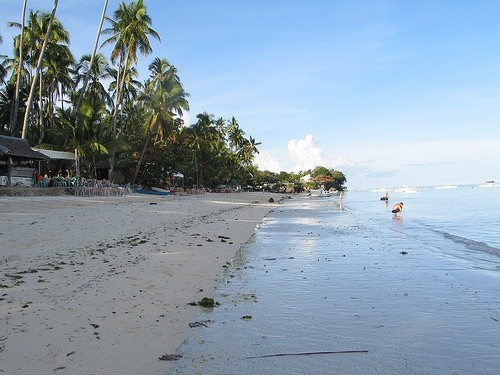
[37,174,122,197]
[186,186,231,195]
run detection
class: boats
[372,187,422,193]
[311,188,332,197]
[435,185,457,189]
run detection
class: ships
[478,181,495,188]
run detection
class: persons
[385,192,390,201]
[37,170,70,187]
[391,202,403,213]
[187,188,207,195]
[340,191,343,202]
